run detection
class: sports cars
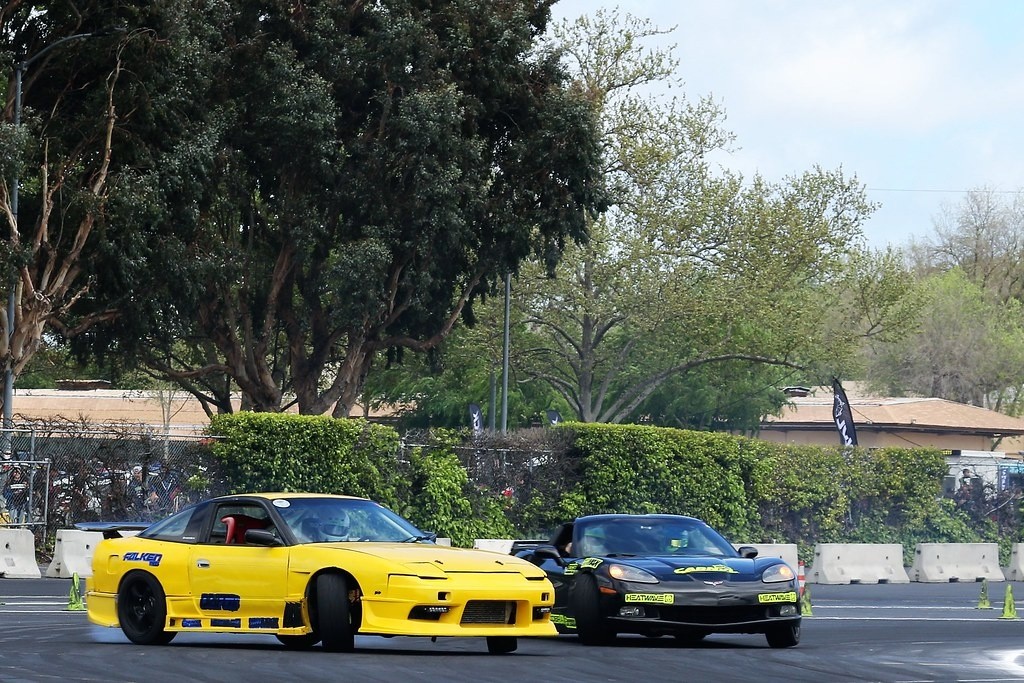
[85,491,561,654]
[511,513,803,650]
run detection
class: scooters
[0,445,212,541]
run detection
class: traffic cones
[975,579,994,609]
[62,571,87,611]
[798,561,806,598]
[997,583,1022,619]
[798,588,814,618]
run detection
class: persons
[944,468,1024,526]
[564,525,611,556]
[309,507,349,541]
[0,450,180,529]
[659,527,688,554]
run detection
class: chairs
[221,515,267,544]
[548,524,586,548]
[301,518,319,542]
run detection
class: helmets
[317,508,350,540]
[662,527,688,548]
[583,527,608,543]
